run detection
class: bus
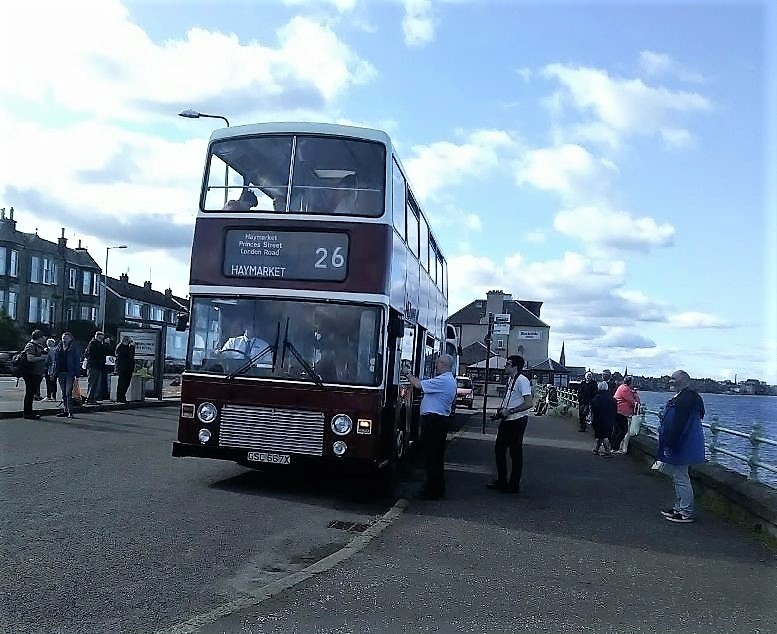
[168,121,450,486]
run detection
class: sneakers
[660,507,695,523]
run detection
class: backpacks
[10,347,31,387]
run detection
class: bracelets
[405,371,411,377]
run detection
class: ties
[244,340,252,360]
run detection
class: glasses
[505,362,512,366]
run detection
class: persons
[113,336,136,403]
[23,330,80,419]
[655,370,707,523]
[214,319,272,364]
[85,331,110,404]
[577,369,641,457]
[223,189,258,210]
[529,383,557,416]
[401,354,457,500]
[488,355,533,493]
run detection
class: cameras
[490,408,507,422]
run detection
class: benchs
[546,397,565,415]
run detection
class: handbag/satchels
[628,402,643,437]
[71,380,80,399]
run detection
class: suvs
[453,376,474,410]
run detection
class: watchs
[509,408,514,414]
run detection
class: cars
[0,351,21,377]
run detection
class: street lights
[100,245,128,343]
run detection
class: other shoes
[84,399,98,405]
[577,424,625,458]
[34,397,44,401]
[413,489,445,500]
[41,396,56,402]
[115,399,127,404]
[494,479,521,494]
[529,407,543,416]
[23,413,40,420]
[56,412,73,419]
